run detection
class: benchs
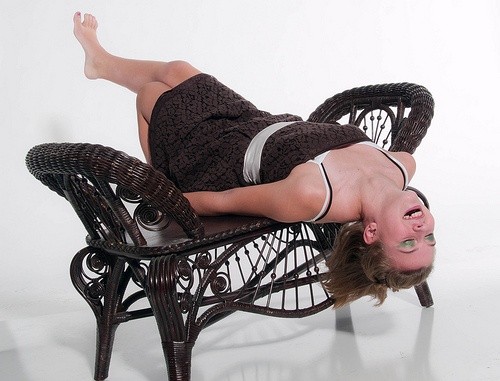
[25,82,434,381]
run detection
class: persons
[73,11,436,310]
